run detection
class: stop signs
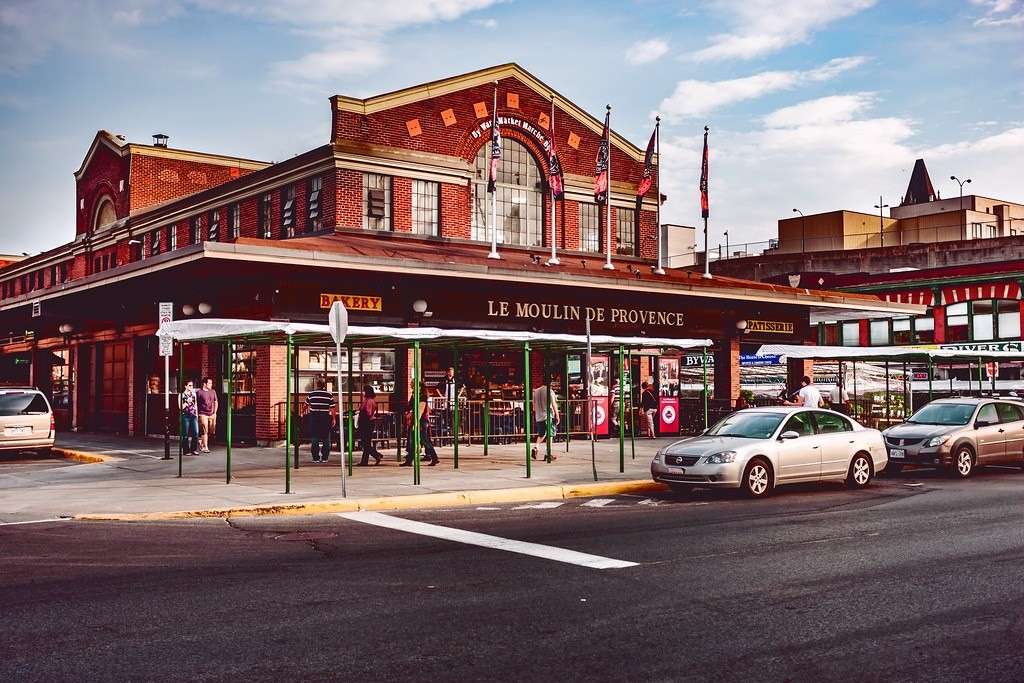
[986,362,1000,378]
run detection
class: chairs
[791,422,808,436]
[950,411,965,423]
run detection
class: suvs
[0,386,56,458]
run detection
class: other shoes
[357,463,363,465]
[193,450,199,455]
[312,459,319,462]
[375,454,384,465]
[323,458,329,462]
[544,455,557,461]
[203,449,210,453]
[531,448,537,459]
[186,453,192,456]
[421,454,431,462]
[197,440,203,451]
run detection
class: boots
[427,449,439,466]
[399,455,414,467]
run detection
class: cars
[882,396,1024,478]
[650,406,889,500]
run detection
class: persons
[641,381,660,439]
[611,378,626,434]
[783,375,824,408]
[398,381,439,466]
[196,376,219,453]
[355,385,384,465]
[588,364,608,386]
[301,378,337,463]
[531,375,560,461]
[828,382,852,415]
[660,362,679,396]
[436,367,466,398]
[176,380,199,456]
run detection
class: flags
[487,108,502,193]
[548,115,565,202]
[699,137,711,219]
[635,124,656,197]
[595,116,608,204]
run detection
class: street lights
[950,176,972,241]
[723,231,729,259]
[688,244,697,264]
[793,208,804,252]
[874,196,889,247]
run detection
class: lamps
[581,260,586,268]
[651,266,655,275]
[198,301,219,315]
[183,304,194,315]
[635,269,641,279]
[687,271,692,278]
[413,299,427,327]
[59,322,79,345]
[626,265,632,273]
[530,254,542,264]
